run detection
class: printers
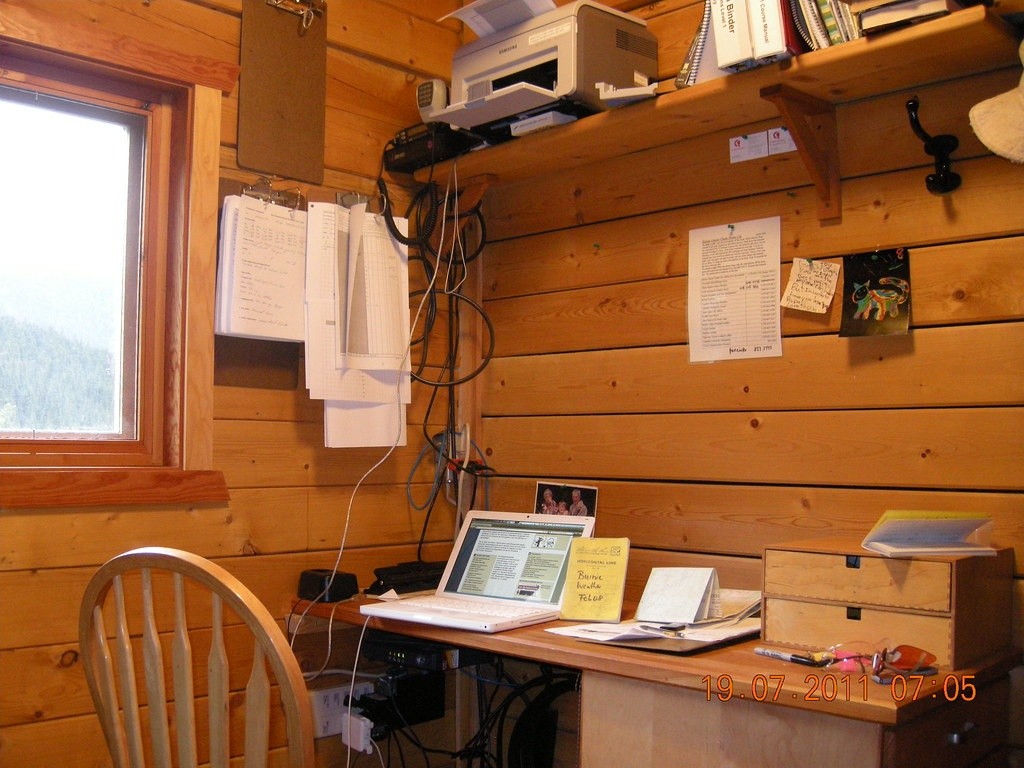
[430,0,660,141]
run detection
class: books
[862,510,996,557]
[675,0,857,89]
[851,0,962,36]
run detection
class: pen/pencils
[639,624,685,638]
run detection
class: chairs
[79,546,315,768]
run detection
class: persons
[538,488,587,516]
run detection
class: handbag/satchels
[364,561,446,596]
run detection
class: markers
[754,647,817,667]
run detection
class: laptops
[359,510,596,634]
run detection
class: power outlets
[307,681,375,739]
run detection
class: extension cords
[304,681,374,738]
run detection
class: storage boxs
[709,0,756,75]
[761,536,1014,674]
[748,0,802,66]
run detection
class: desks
[293,587,1024,768]
